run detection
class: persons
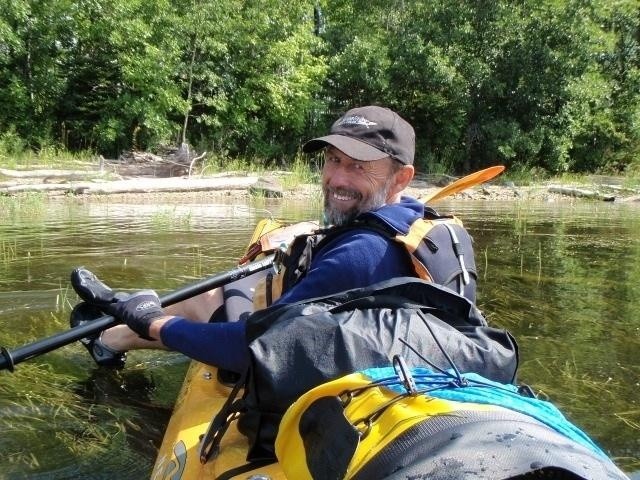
[69,103,479,372]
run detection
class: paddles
[0,165,505,372]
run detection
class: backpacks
[311,206,478,305]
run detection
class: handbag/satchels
[232,277,520,462]
[275,366,632,480]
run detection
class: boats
[151,217,629,480]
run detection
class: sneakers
[71,267,130,315]
[70,302,127,370]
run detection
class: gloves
[110,289,168,341]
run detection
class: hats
[302,104,415,165]
